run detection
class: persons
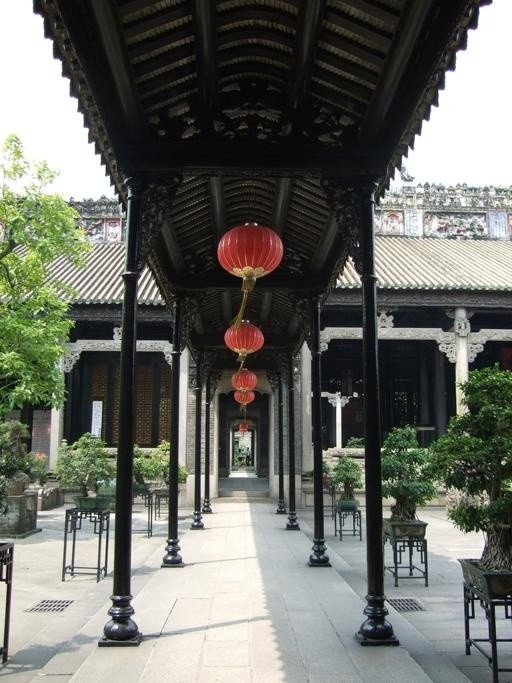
[244,446,252,465]
[237,448,243,465]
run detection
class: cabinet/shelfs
[0,543,14,665]
[132,491,153,538]
[155,495,168,521]
[461,584,512,682]
[334,509,362,541]
[62,507,111,582]
[383,535,428,587]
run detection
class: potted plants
[56,432,117,510]
[330,456,364,510]
[380,425,436,538]
[133,444,160,492]
[153,440,188,496]
[425,362,512,601]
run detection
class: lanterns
[231,388,258,413]
[215,220,287,330]
[239,421,249,436]
[229,365,260,402]
[222,317,264,373]
